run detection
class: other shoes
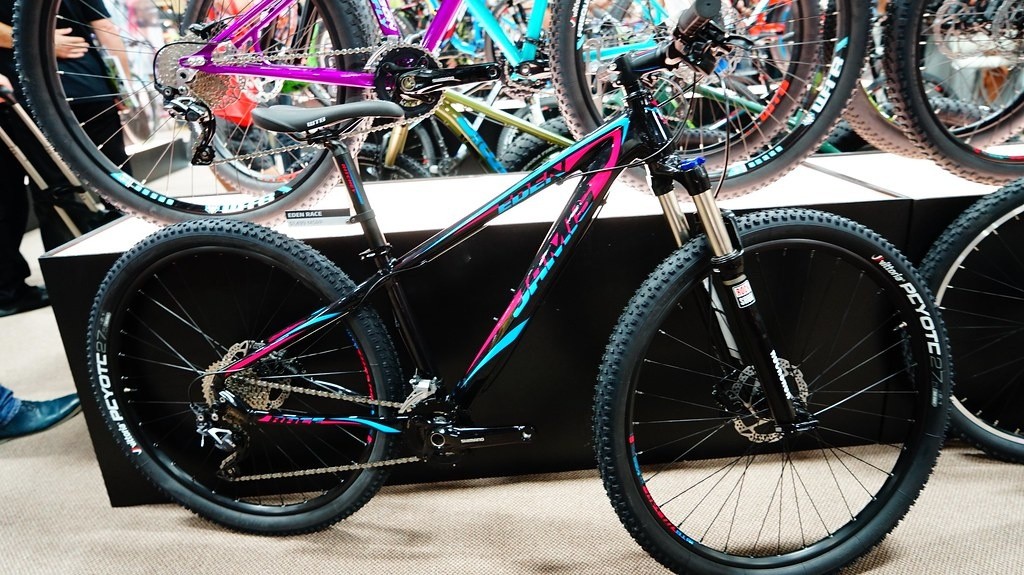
[0,285,51,317]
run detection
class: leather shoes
[0,393,82,439]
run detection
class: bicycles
[11,0,1024,575]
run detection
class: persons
[0,0,132,442]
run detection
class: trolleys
[0,84,126,240]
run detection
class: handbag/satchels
[89,33,140,110]
